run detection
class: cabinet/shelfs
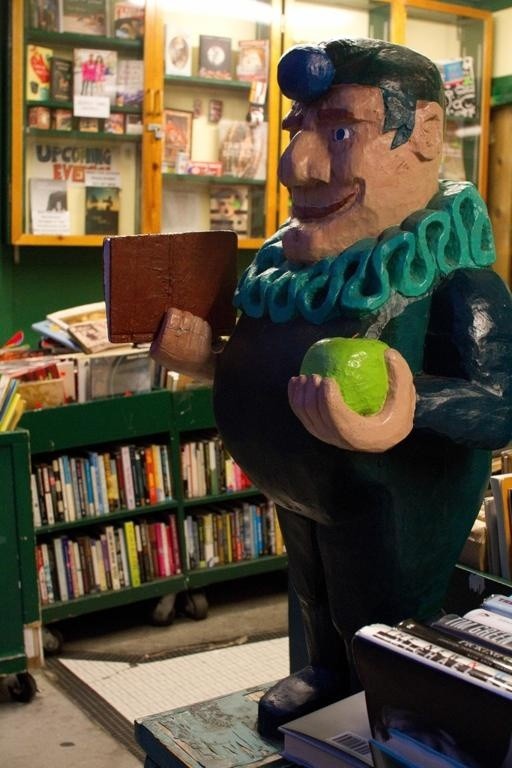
[0,417,44,704]
[279,0,493,246]
[22,390,288,653]
[8,1,283,246]
[448,554,512,618]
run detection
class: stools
[133,680,285,768]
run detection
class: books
[100,231,238,344]
[278,443,510,767]
[23,1,271,236]
[2,302,289,603]
[440,57,474,116]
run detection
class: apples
[301,335,390,415]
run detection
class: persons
[146,36,512,738]
